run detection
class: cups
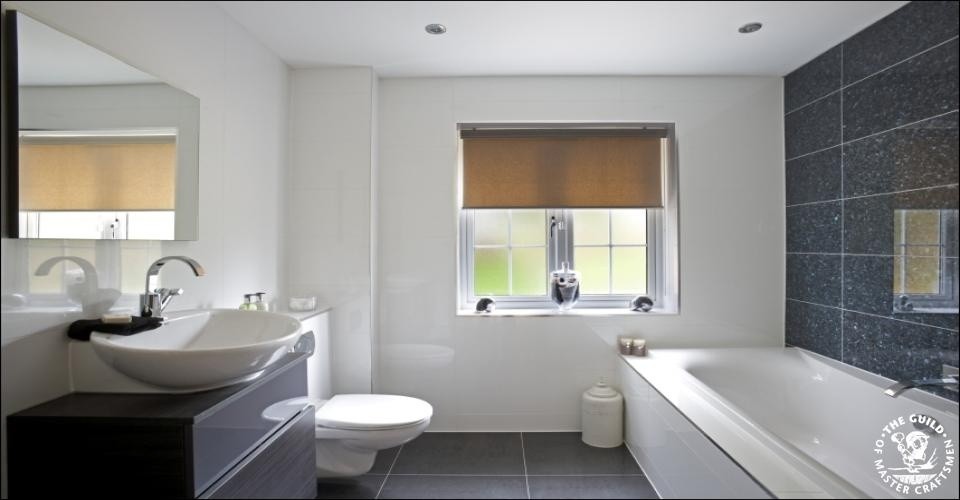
[634,339,646,357]
[621,338,632,354]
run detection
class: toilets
[262,396,309,420]
[312,388,434,477]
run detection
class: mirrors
[3,8,205,243]
[890,207,959,315]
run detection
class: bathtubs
[620,339,960,500]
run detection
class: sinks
[85,301,303,390]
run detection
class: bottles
[550,261,580,309]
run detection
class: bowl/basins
[287,297,318,312]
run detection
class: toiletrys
[239,291,271,313]
[100,313,134,324]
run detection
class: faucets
[139,251,208,320]
[883,361,959,402]
[29,253,123,320]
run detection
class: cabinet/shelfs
[6,353,321,500]
